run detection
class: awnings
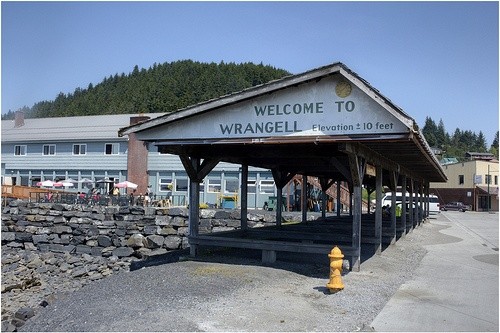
[476,186,498,196]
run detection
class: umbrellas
[76,178,94,190]
[98,178,113,194]
[113,180,138,195]
[36,179,56,189]
[58,178,78,189]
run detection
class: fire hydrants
[326,245,350,292]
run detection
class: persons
[297,197,323,212]
[75,191,101,204]
[113,186,120,196]
[129,190,151,206]
[379,204,402,218]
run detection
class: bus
[382,191,439,214]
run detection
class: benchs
[193,214,423,269]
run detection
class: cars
[440,202,469,212]
